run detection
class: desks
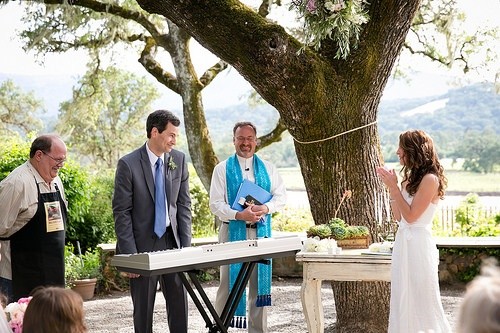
[295,246,392,333]
[436,237,500,248]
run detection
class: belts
[223,221,257,229]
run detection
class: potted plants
[65,211,103,300]
[308,218,370,249]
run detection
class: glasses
[41,152,67,163]
[235,136,257,143]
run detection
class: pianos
[111,235,302,277]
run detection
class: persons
[378,130,451,333]
[453,256,500,333]
[20,284,86,332]
[0,134,70,313]
[208,121,287,333]
[112,111,191,333]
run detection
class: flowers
[4,296,34,333]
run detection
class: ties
[154,158,167,238]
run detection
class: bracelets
[389,199,395,202]
